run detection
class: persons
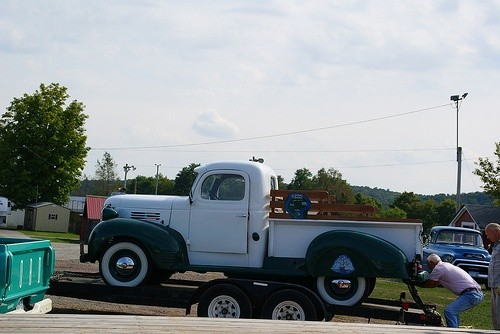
[485,223,500,330]
[419,253,483,328]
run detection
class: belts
[460,288,481,296]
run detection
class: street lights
[155,164,162,195]
[450,92,468,215]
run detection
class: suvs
[423,226,492,291]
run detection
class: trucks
[80,161,422,321]
[1,238,55,316]
